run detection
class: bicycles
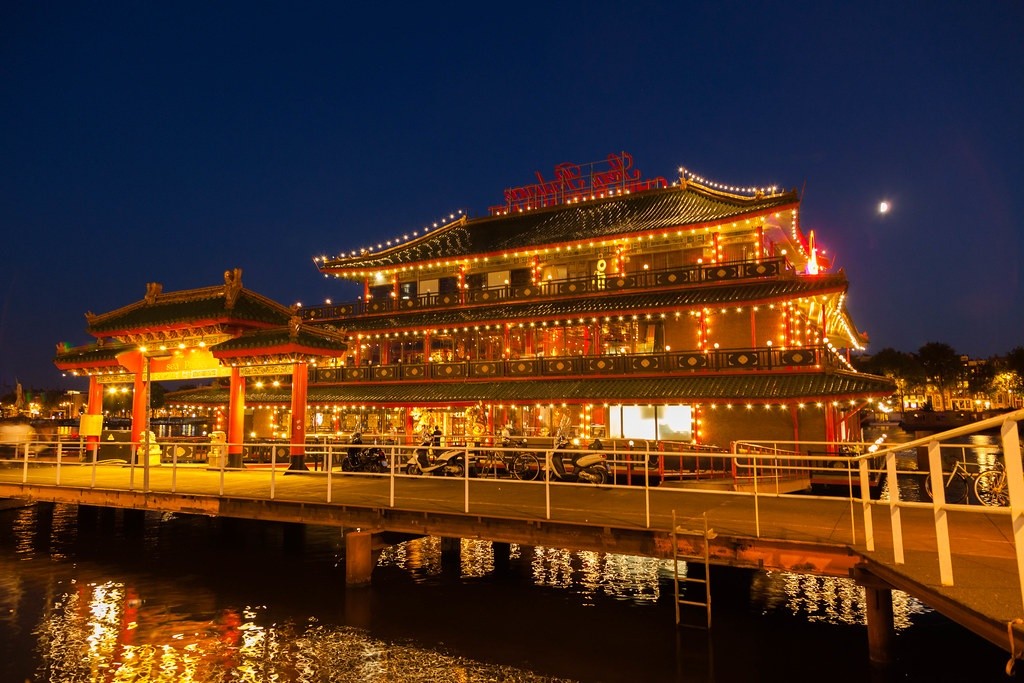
[479,437,540,481]
[923,452,1010,508]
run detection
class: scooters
[341,432,387,473]
[541,434,612,485]
[405,432,466,478]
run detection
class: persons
[389,423,397,442]
[432,426,442,446]
[501,424,510,444]
[419,425,432,438]
[922,402,932,410]
